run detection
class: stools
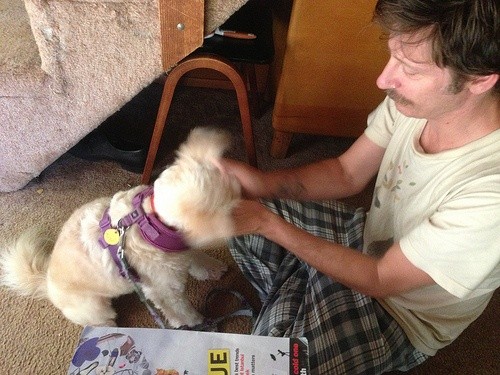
[137,0,273,185]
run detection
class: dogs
[1,125,245,328]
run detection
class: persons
[223,0,500,375]
